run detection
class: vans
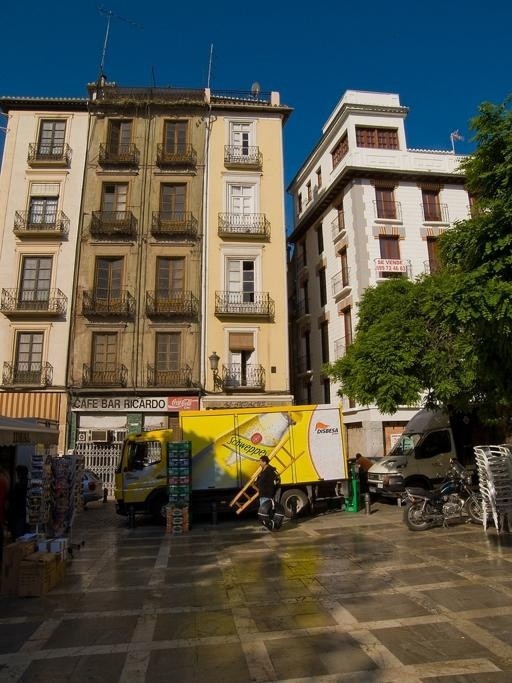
[365,398,512,504]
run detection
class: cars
[81,467,107,507]
[316,454,391,502]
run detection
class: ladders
[230,437,305,515]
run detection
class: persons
[355,452,374,489]
[254,454,279,531]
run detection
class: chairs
[473,445,512,537]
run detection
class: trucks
[109,397,351,524]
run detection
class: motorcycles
[398,455,497,531]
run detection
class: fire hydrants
[101,487,109,504]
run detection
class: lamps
[209,352,220,392]
